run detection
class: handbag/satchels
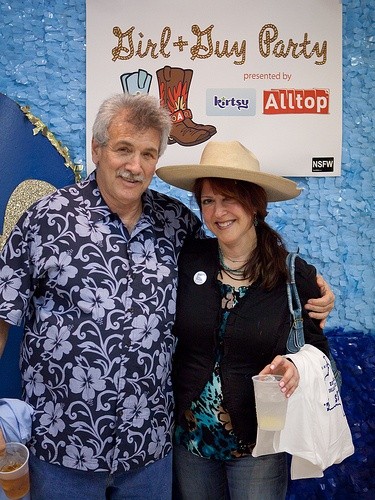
[286,251,343,394]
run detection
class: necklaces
[217,247,254,281]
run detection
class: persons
[1,92,335,500]
[156,141,329,500]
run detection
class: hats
[155,139,301,203]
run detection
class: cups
[251,374,288,432]
[0,441,30,500]
